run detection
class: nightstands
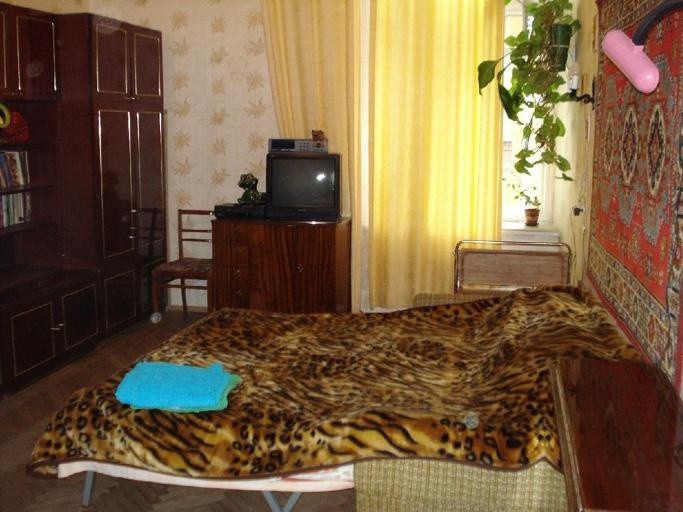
[548,359,682,511]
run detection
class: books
[0,148,32,230]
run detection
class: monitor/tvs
[265,152,340,221]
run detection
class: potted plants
[478,1,581,182]
[501,174,541,226]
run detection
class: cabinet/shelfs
[0,99,52,236]
[100,256,170,334]
[212,217,351,315]
[51,102,167,263]
[1,2,57,101]
[0,268,101,396]
[57,13,164,102]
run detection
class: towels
[115,361,242,414]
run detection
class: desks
[503,220,562,253]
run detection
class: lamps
[600,0,683,95]
[567,61,595,110]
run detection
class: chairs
[452,239,571,294]
[151,209,215,324]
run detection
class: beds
[26,284,645,512]
